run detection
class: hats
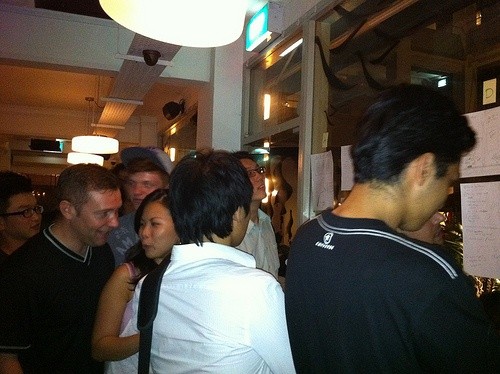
[119,146,174,176]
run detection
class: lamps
[67,96,104,166]
[71,76,120,154]
[98,0,248,49]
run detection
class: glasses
[3,205,44,218]
[248,166,267,177]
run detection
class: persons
[229,150,280,280]
[106,145,175,277]
[106,148,298,374]
[284,81,500,374]
[0,170,45,259]
[396,205,448,246]
[111,161,137,216]
[0,164,124,374]
[90,186,181,362]
